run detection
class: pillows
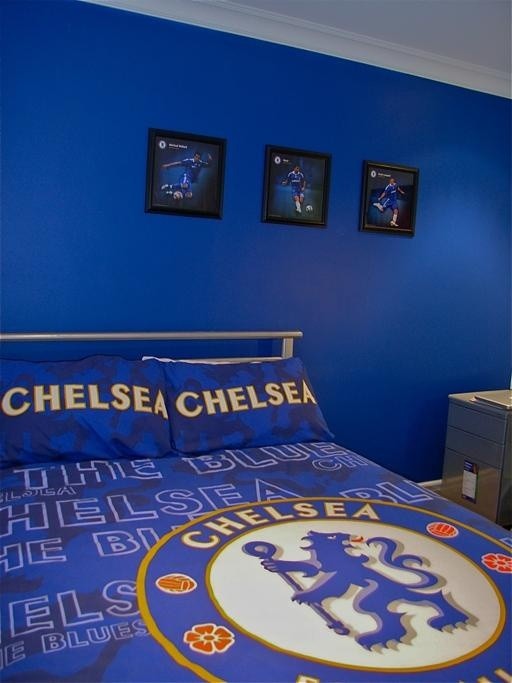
[0,359,174,461]
[165,353,335,451]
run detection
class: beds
[0,330,512,682]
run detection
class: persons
[282,165,306,214]
[161,151,213,202]
[372,178,405,227]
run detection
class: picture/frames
[145,127,228,221]
[261,144,331,229]
[360,159,420,237]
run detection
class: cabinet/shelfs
[440,389,512,525]
